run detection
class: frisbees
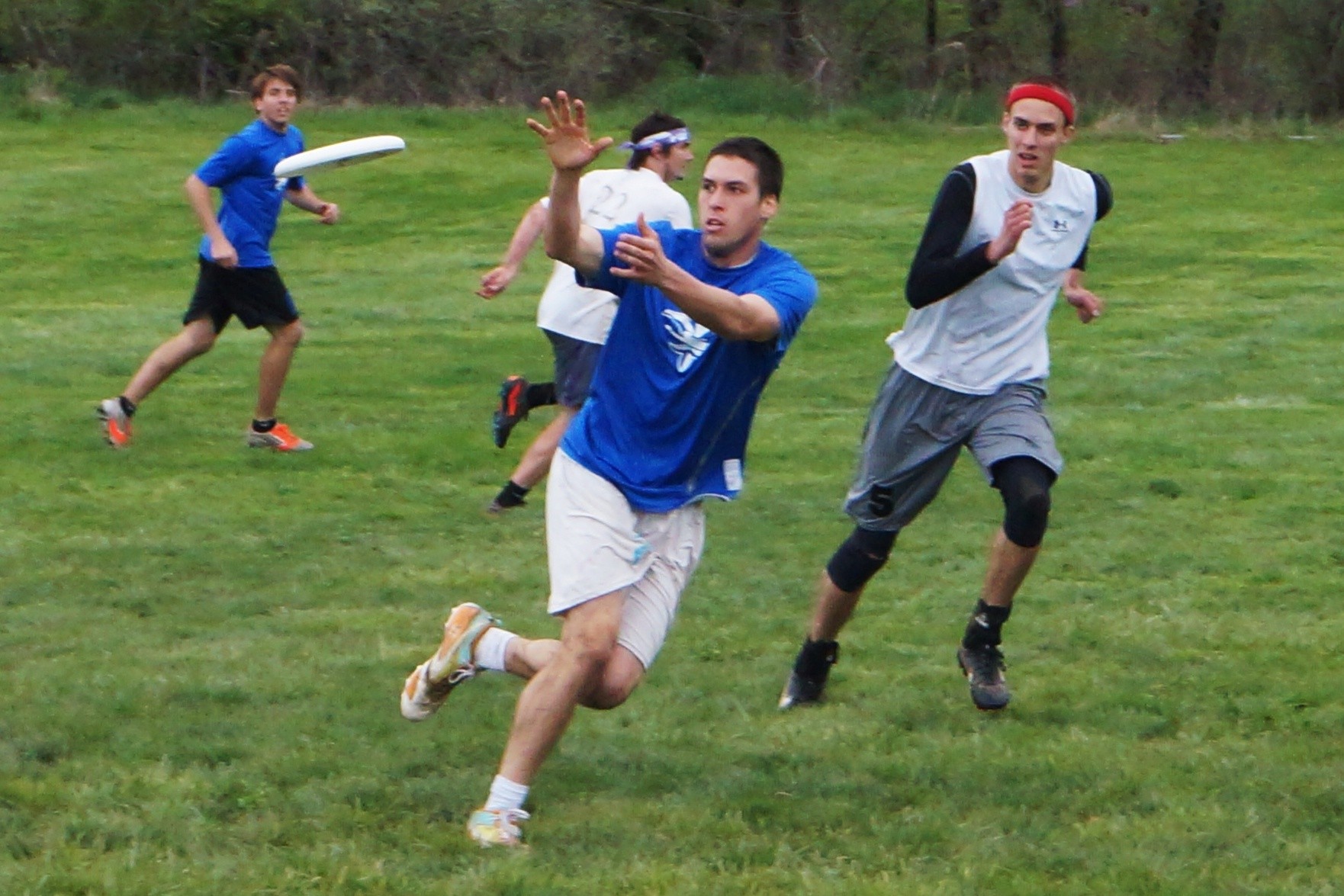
[274,135,405,177]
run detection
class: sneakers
[95,396,133,449]
[492,375,529,447]
[485,499,505,514]
[246,419,315,450]
[465,802,531,850]
[400,601,508,719]
[955,637,1011,710]
[777,638,841,707]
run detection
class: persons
[471,106,694,515]
[779,76,1113,713]
[92,63,341,452]
[398,89,819,840]
